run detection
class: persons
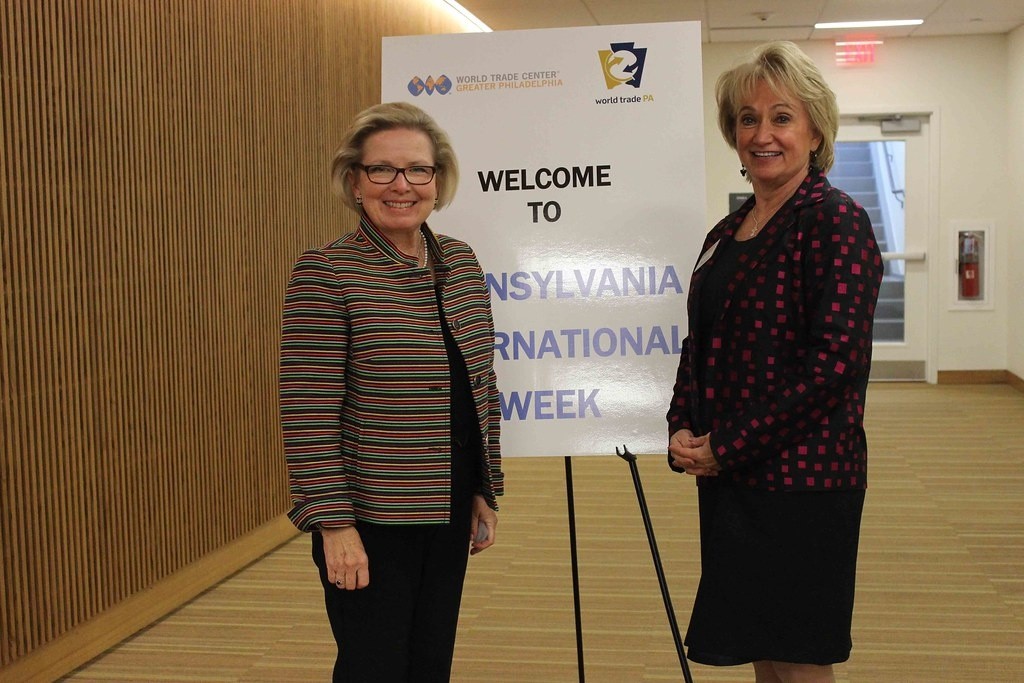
[667,40,884,683]
[278,102,504,683]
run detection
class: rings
[336,581,344,585]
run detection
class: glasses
[353,162,438,185]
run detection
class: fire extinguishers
[959,232,980,297]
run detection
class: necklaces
[750,184,797,236]
[417,230,429,269]
